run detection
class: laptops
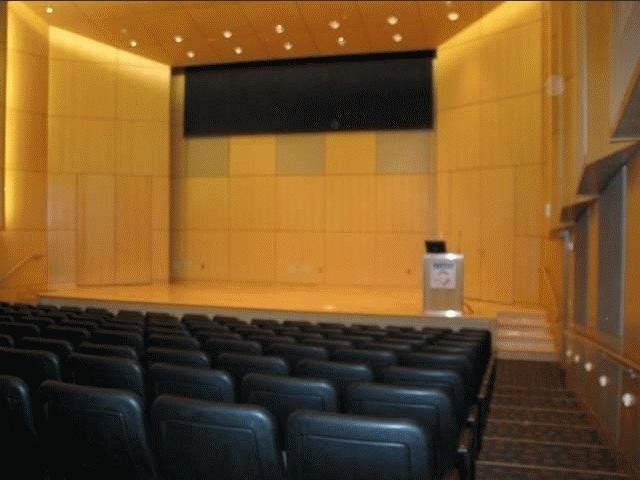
[425,239,446,253]
